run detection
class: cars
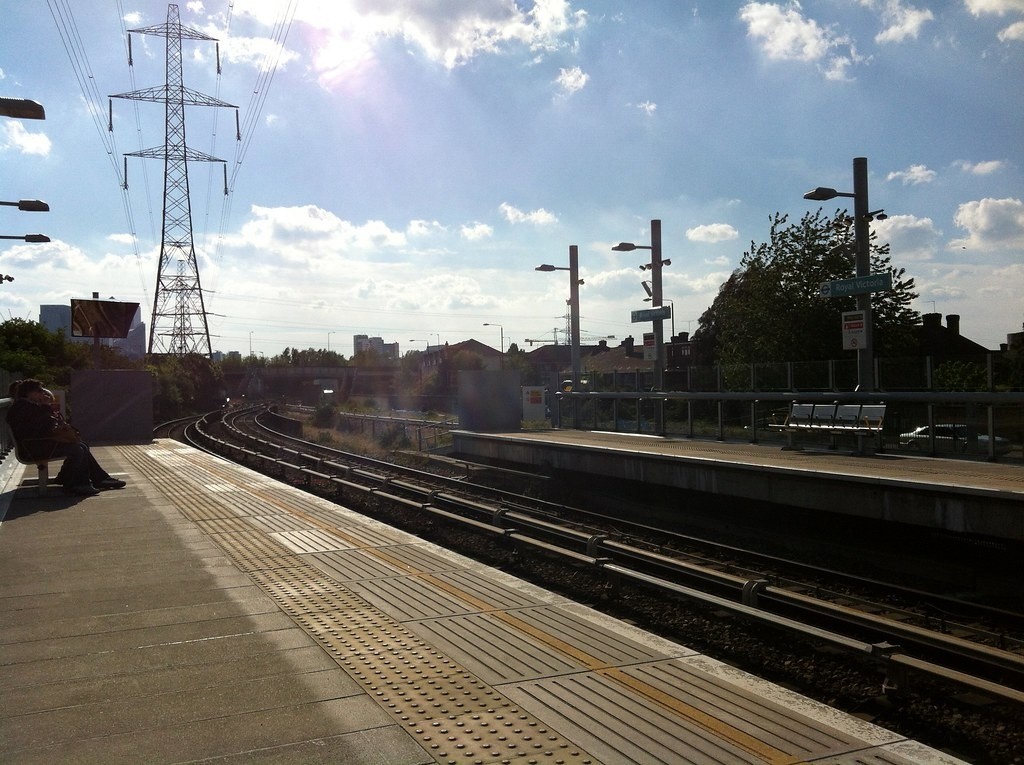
[899,424,1013,459]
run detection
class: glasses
[34,388,44,392]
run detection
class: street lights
[534,245,584,430]
[250,331,253,357]
[612,219,672,436]
[804,157,888,449]
[411,339,430,375]
[643,298,676,392]
[328,332,334,353]
[483,323,504,388]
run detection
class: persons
[6,379,127,494]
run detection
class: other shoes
[92,478,127,488]
[82,485,101,496]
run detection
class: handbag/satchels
[47,413,81,442]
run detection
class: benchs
[769,404,887,457]
[4,422,68,499]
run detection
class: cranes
[524,327,616,346]
